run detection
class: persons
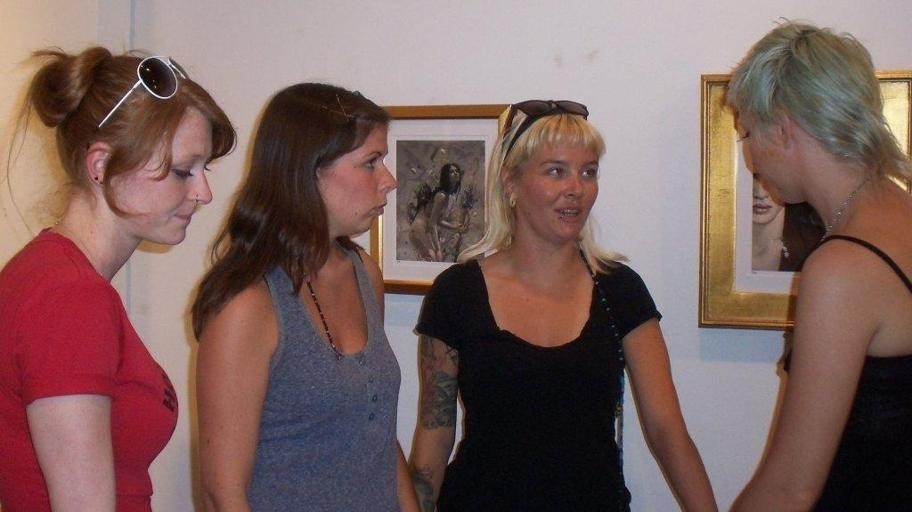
[402,102,719,511]
[410,163,472,262]
[3,43,234,512]
[751,170,814,271]
[727,23,912,510]
[195,82,424,511]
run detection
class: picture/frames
[368,103,510,295]
[698,72,911,331]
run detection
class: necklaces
[819,167,875,239]
[298,261,340,353]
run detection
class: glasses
[500,99,590,153]
[95,54,191,130]
